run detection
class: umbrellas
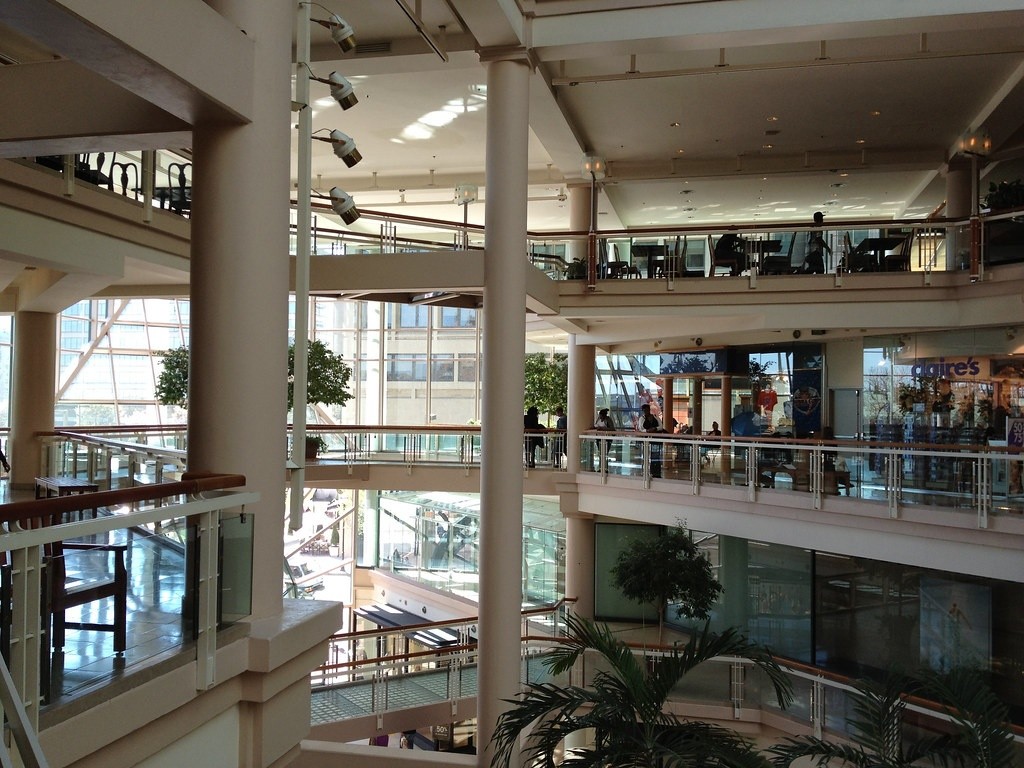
[731,412,768,437]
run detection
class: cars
[285,562,325,593]
[310,489,342,514]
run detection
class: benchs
[34,476,99,525]
[0,541,130,656]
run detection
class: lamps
[455,184,478,249]
[300,2,358,53]
[584,155,607,232]
[313,128,363,168]
[958,134,991,216]
[298,60,359,112]
[310,188,361,225]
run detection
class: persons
[553,408,567,467]
[595,410,615,473]
[821,427,854,487]
[638,404,663,478]
[994,406,1010,440]
[715,225,754,276]
[678,425,693,435]
[524,406,538,468]
[370,735,389,747]
[806,212,833,274]
[1009,460,1024,494]
[707,422,721,448]
[400,729,416,750]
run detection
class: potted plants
[289,436,329,461]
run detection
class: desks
[745,239,782,276]
[131,185,193,218]
[632,244,668,279]
[854,238,906,273]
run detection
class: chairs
[596,229,913,281]
[166,163,193,219]
